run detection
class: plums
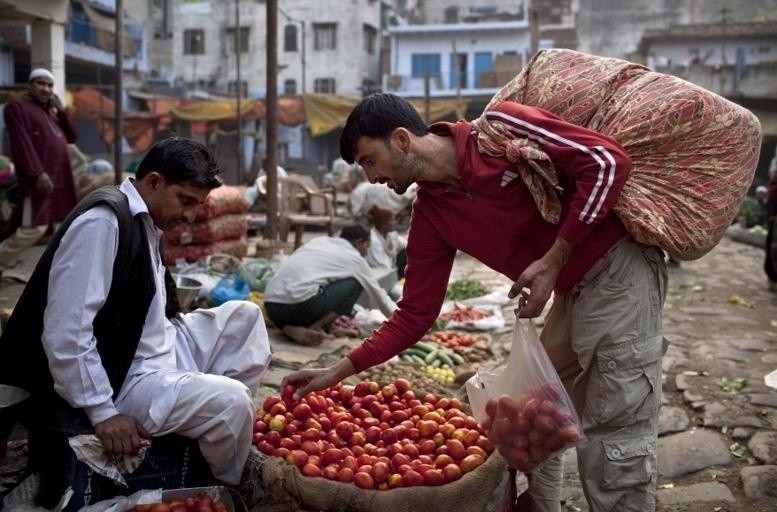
[132,495,228,512]
[480,381,587,474]
[252,379,493,491]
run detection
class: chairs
[258,172,333,251]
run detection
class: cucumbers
[401,341,465,367]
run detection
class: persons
[263,222,397,346]
[335,180,419,300]
[0,67,79,264]
[281,92,665,512]
[1,137,273,487]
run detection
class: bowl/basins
[169,272,203,314]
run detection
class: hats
[28,68,55,83]
